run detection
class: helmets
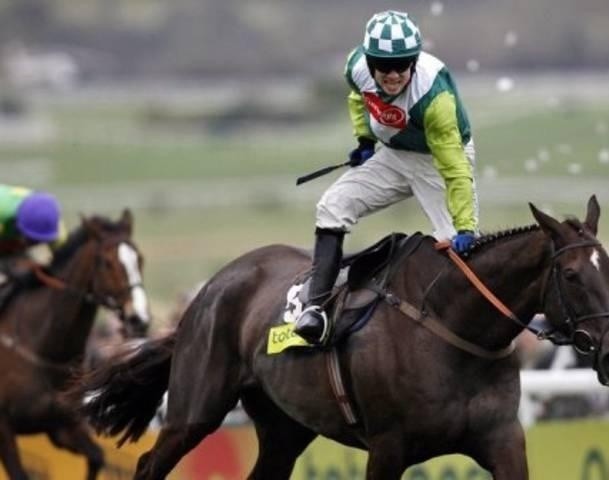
[362,11,421,58]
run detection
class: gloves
[453,231,475,252]
[351,144,374,165]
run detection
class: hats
[17,195,59,241]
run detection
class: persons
[296,10,477,343]
[1,185,68,287]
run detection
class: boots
[294,227,344,337]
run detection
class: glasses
[374,61,411,73]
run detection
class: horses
[66,193,609,479]
[0,207,152,480]
